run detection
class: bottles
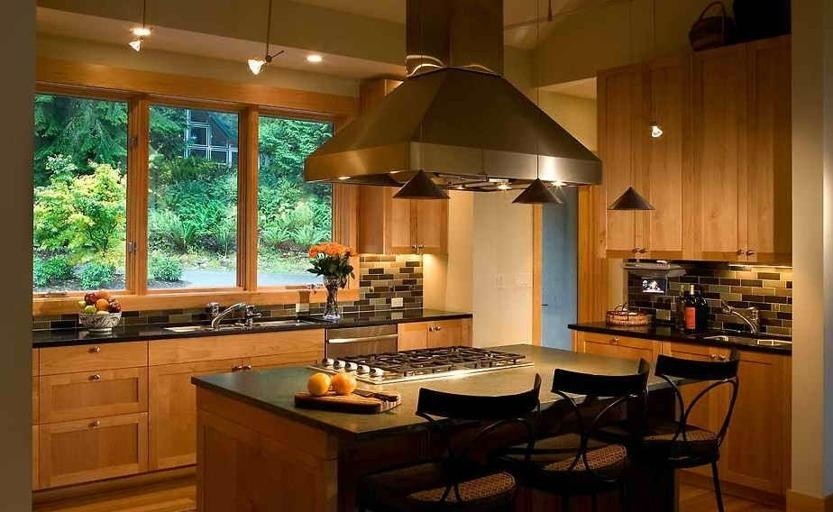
[683,284,699,334]
[675,283,685,331]
[696,290,709,333]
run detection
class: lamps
[247,1,284,76]
[131,1,149,50]
[392,3,449,201]
[512,1,564,206]
[610,1,655,212]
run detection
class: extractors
[304,0,603,192]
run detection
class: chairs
[358,374,542,512]
[599,350,740,512]
[502,359,655,512]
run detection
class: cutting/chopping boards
[295,384,403,415]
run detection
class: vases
[320,275,340,321]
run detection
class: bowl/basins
[79,313,123,336]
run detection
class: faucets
[244,305,262,327]
[722,306,760,334]
[211,303,242,328]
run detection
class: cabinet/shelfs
[147,330,326,473]
[360,190,447,258]
[572,334,790,497]
[594,46,792,263]
[399,319,473,353]
[30,342,148,494]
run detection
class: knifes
[350,388,398,404]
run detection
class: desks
[190,345,704,512]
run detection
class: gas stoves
[322,346,531,385]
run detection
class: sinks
[702,335,792,348]
[254,319,314,327]
[162,325,211,333]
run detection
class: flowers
[311,243,352,277]
